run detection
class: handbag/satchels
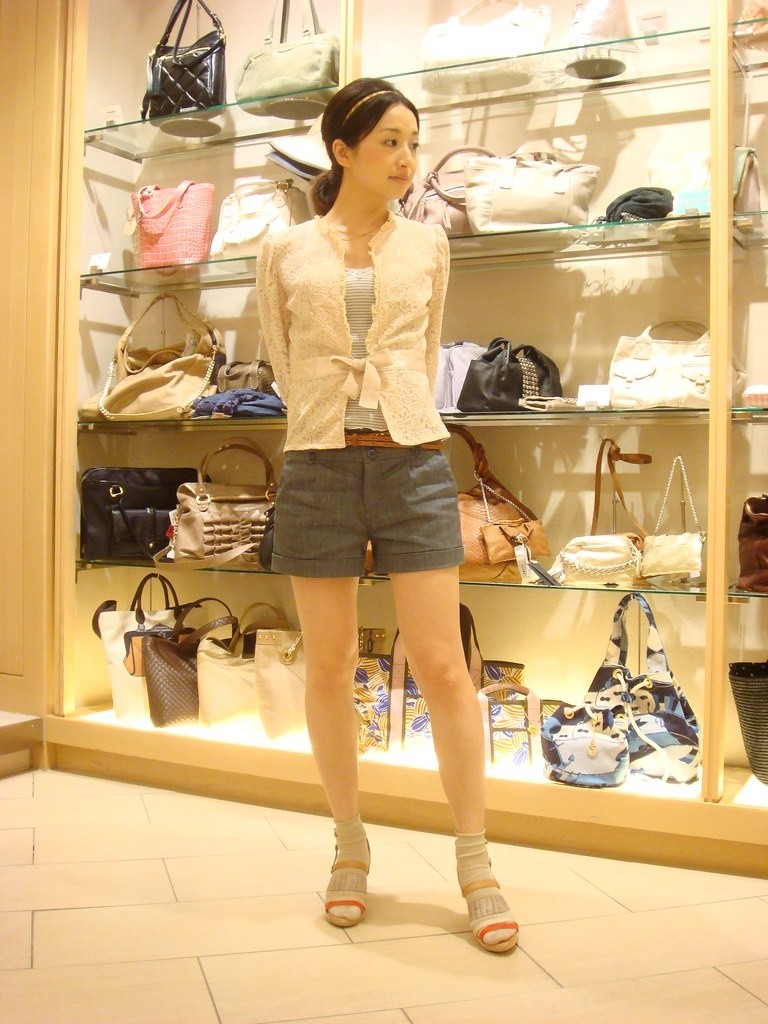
[91,573,701,792]
[420,0,552,95]
[124,181,214,276]
[79,437,275,569]
[396,144,601,253]
[727,660,768,785]
[139,0,226,127]
[647,143,765,237]
[235,0,340,118]
[77,290,288,420]
[735,494,768,593]
[550,438,652,582]
[434,317,711,413]
[638,454,708,583]
[210,177,314,274]
[445,424,551,567]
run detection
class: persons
[253,77,520,955]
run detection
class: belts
[346,433,445,450]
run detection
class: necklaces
[335,221,385,238]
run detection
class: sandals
[455,858,519,952]
[325,828,371,927]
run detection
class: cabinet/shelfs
[41,0,768,879]
